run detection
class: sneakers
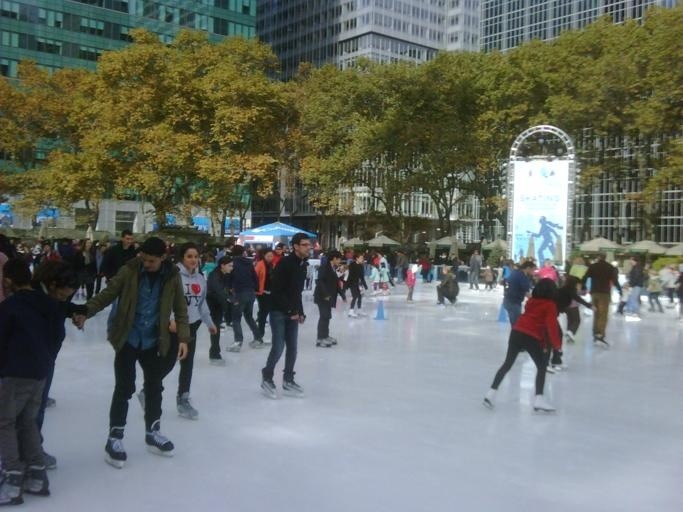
[349,309,365,317]
[283,381,302,392]
[227,340,264,350]
[486,389,497,405]
[42,454,57,469]
[593,335,608,346]
[263,380,276,393]
[209,351,221,359]
[316,337,336,347]
[535,396,555,410]
[566,330,575,342]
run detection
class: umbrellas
[240,222,317,251]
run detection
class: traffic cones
[496,302,508,322]
[373,300,387,320]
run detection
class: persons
[562,252,682,347]
[30,261,88,470]
[137,241,218,420]
[313,246,392,348]
[73,238,192,472]
[482,278,561,413]
[0,258,66,505]
[1,230,314,365]
[394,248,560,328]
[259,233,310,398]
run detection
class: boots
[176,392,197,416]
[105,425,127,460]
[25,467,52,495]
[1,471,24,505]
[145,419,173,450]
